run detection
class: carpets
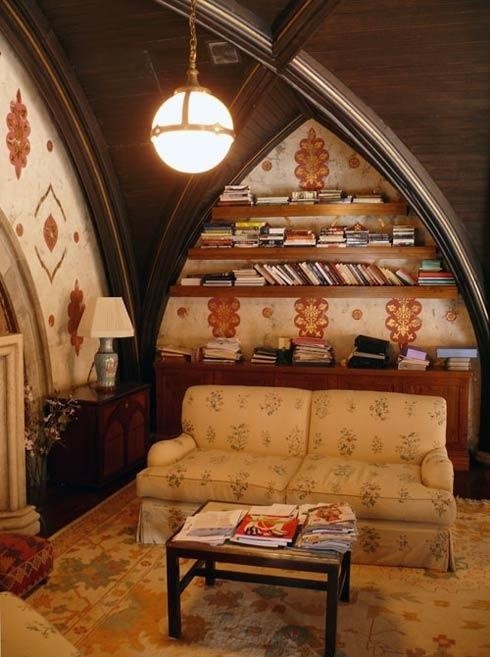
[17,482,487,657]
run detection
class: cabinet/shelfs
[145,358,475,472]
[168,197,461,300]
[46,378,151,497]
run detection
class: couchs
[135,383,457,574]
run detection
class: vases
[26,449,50,513]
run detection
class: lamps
[75,296,137,389]
[149,0,236,174]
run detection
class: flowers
[21,380,83,504]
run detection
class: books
[251,347,280,364]
[158,345,192,356]
[172,496,357,552]
[436,346,478,371]
[398,347,429,370]
[202,338,241,363]
[178,178,459,288]
[347,334,390,369]
[292,337,332,366]
